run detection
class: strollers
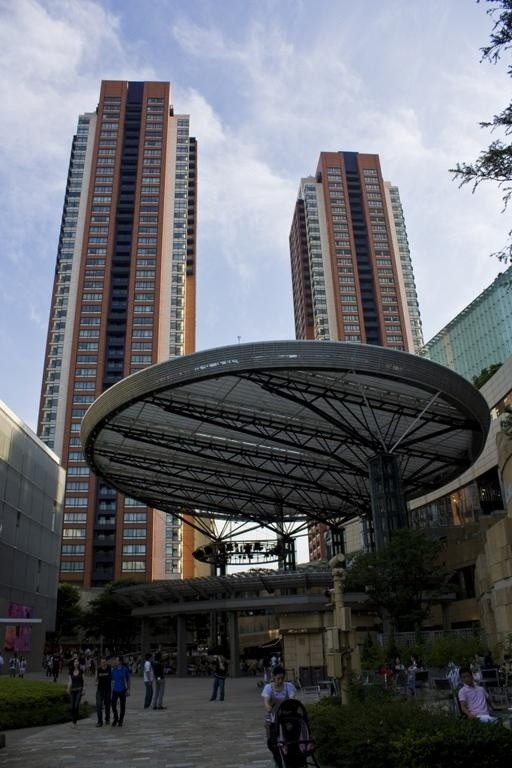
[267,698,320,768]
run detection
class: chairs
[393,668,512,725]
[297,678,318,702]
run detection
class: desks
[314,680,333,698]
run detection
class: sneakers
[144,704,166,711]
[209,697,224,702]
[96,718,123,728]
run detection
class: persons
[261,665,298,753]
[379,650,512,725]
[0,644,283,730]
